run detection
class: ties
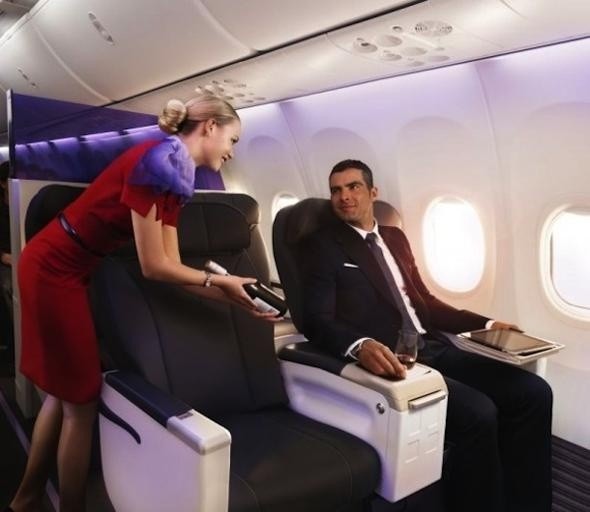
[364,233,425,351]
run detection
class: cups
[395,328,418,370]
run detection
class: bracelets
[203,270,211,288]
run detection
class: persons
[7,94,287,512]
[299,157,556,512]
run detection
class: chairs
[272,195,543,507]
[26,187,382,509]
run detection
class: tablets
[461,326,555,355]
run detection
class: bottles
[205,260,288,319]
